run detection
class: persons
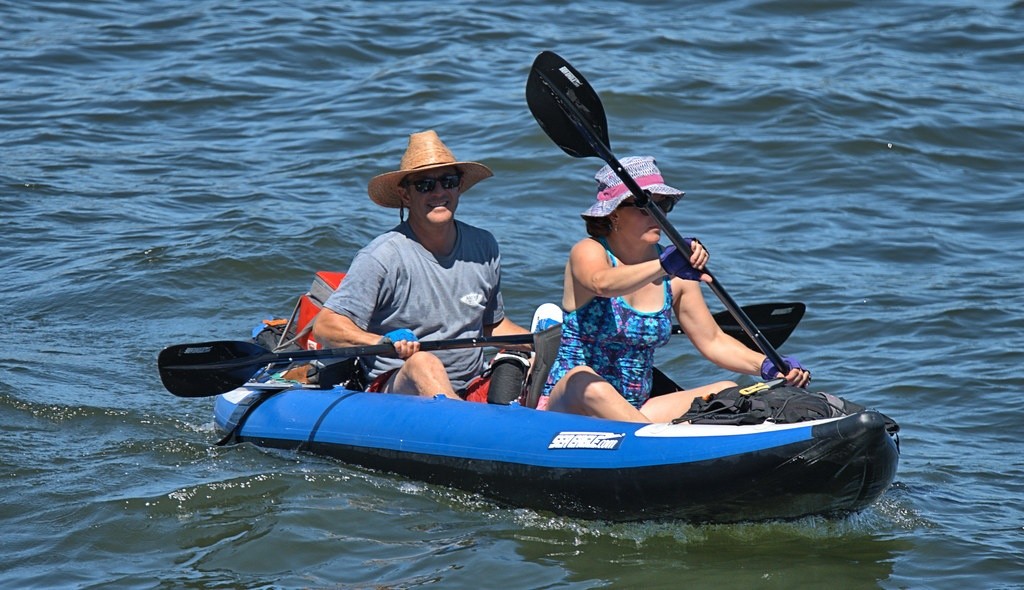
[311,130,533,405]
[536,156,810,424]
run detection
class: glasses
[618,198,674,213]
[401,173,463,193]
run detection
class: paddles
[156,300,807,399]
[522,47,797,379]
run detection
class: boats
[214,322,900,523]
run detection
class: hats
[581,156,687,221]
[368,131,495,208]
